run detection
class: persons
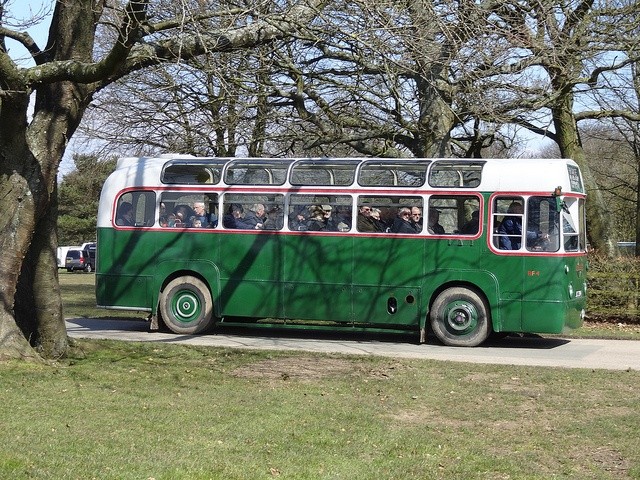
[453,210,481,236]
[192,218,202,227]
[223,203,260,229]
[394,208,418,234]
[263,204,284,230]
[148,203,168,227]
[321,205,332,225]
[244,204,264,229]
[173,204,193,228]
[116,202,134,226]
[498,202,550,251]
[411,207,422,228]
[288,204,309,231]
[383,207,395,233]
[356,201,383,233]
[428,208,445,234]
[370,208,381,221]
[303,205,333,231]
[187,200,218,228]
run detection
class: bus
[94,152,588,348]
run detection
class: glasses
[326,210,330,212]
[404,213,409,215]
[413,214,421,215]
[366,209,372,211]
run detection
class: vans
[64,249,95,272]
